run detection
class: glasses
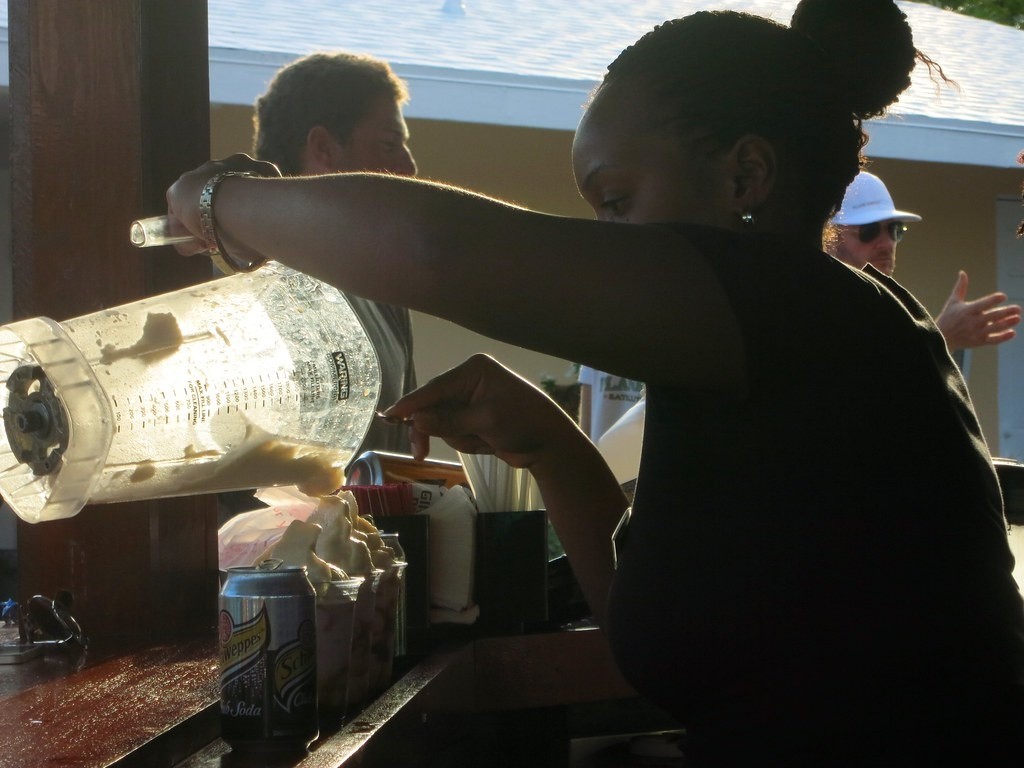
[839,217,908,242]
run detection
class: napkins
[413,484,480,625]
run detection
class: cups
[476,511,549,635]
[312,562,407,737]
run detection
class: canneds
[216,557,320,754]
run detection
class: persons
[215,51,418,458]
[826,170,1021,353]
[577,364,646,504]
[164,2,1024,767]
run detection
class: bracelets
[199,171,271,274]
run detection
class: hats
[831,171,922,226]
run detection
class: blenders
[0,213,412,524]
